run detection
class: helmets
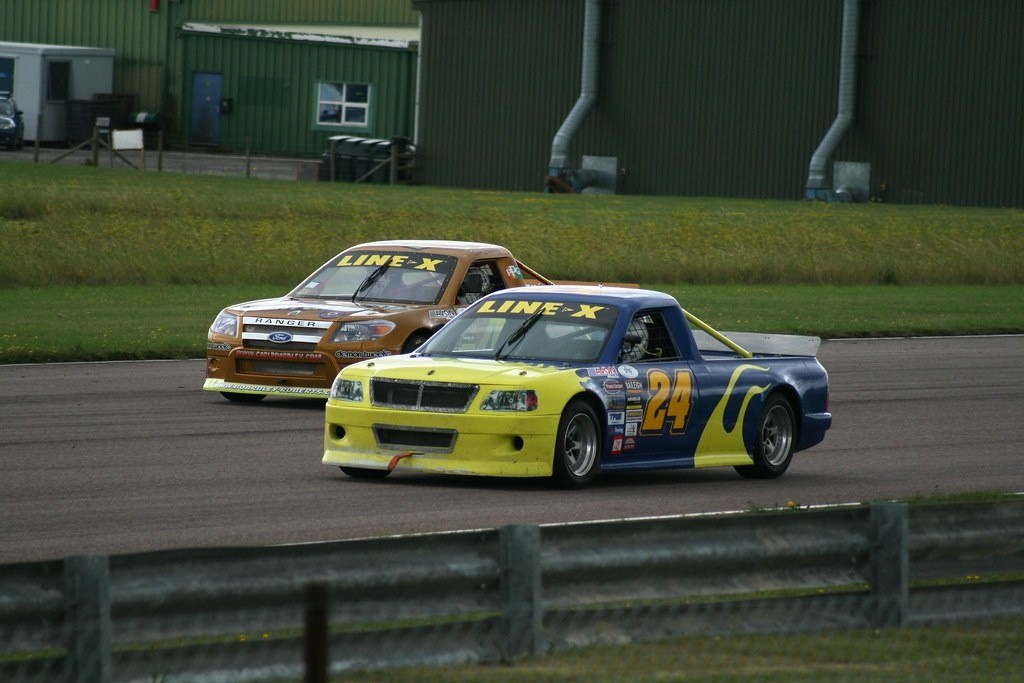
[457,266,489,303]
[591,320,648,361]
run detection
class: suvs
[0,92,24,150]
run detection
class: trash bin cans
[128,111,171,152]
[64,99,121,149]
[321,136,416,186]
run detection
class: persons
[616,320,653,365]
[455,269,492,308]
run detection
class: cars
[203,238,640,401]
[322,286,833,487]
